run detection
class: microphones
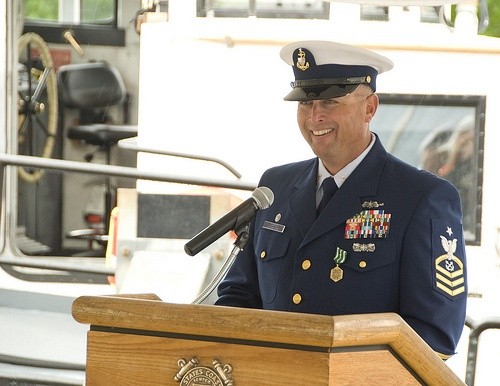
[184,186,275,256]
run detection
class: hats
[280,40,394,101]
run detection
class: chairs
[57,63,139,251]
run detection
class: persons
[213,40,468,362]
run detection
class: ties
[316,177,338,219]
